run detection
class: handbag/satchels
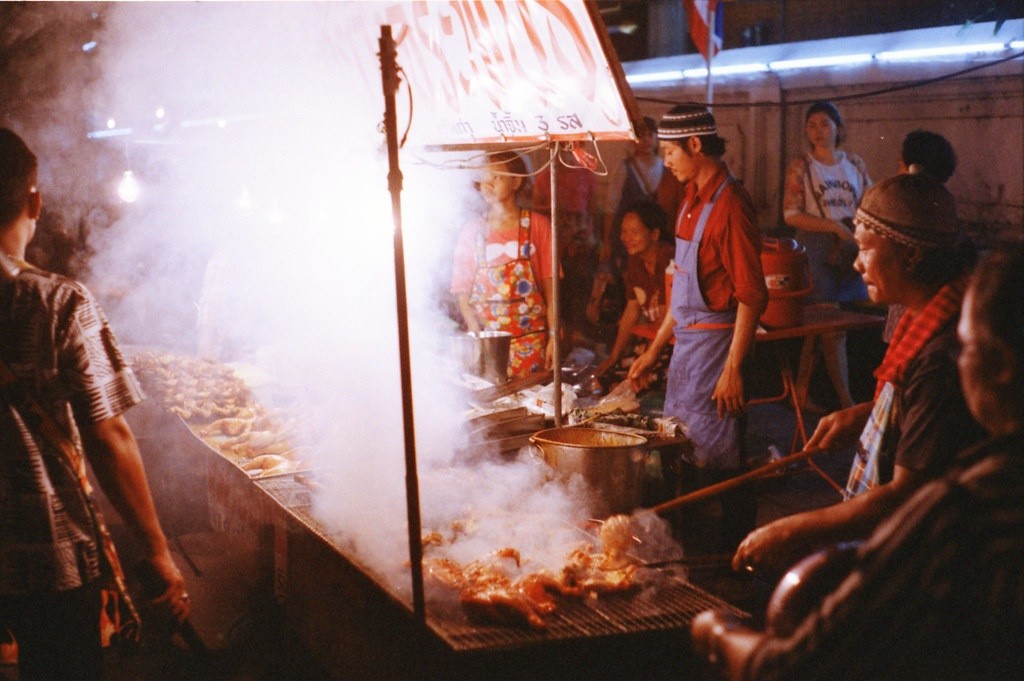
[828,239,859,293]
[101,535,142,647]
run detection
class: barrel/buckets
[468,331,512,385]
[759,238,815,326]
[530,427,649,521]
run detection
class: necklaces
[0,251,25,267]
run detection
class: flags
[681,0,726,66]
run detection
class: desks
[629,303,887,498]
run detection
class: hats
[657,101,717,140]
[855,165,962,253]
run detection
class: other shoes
[790,398,828,413]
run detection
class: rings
[743,565,755,573]
[179,594,187,601]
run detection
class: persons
[727,172,978,579]
[626,102,772,555]
[589,204,679,412]
[20,208,83,281]
[0,454,143,666]
[0,125,193,681]
[897,129,957,183]
[782,102,884,412]
[591,116,664,333]
[445,150,564,386]
[689,242,1024,681]
